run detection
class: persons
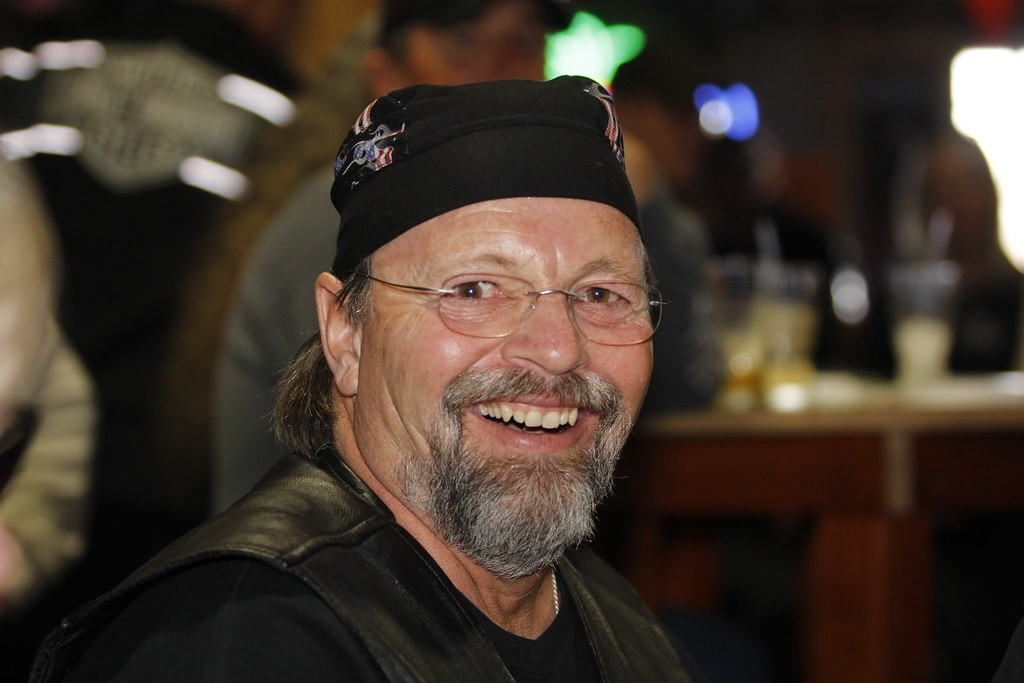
[211,0,727,526]
[24,75,710,683]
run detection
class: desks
[581,374,1023,682]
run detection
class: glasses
[357,273,668,346]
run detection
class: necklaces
[551,565,559,615]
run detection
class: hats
[326,75,640,283]
